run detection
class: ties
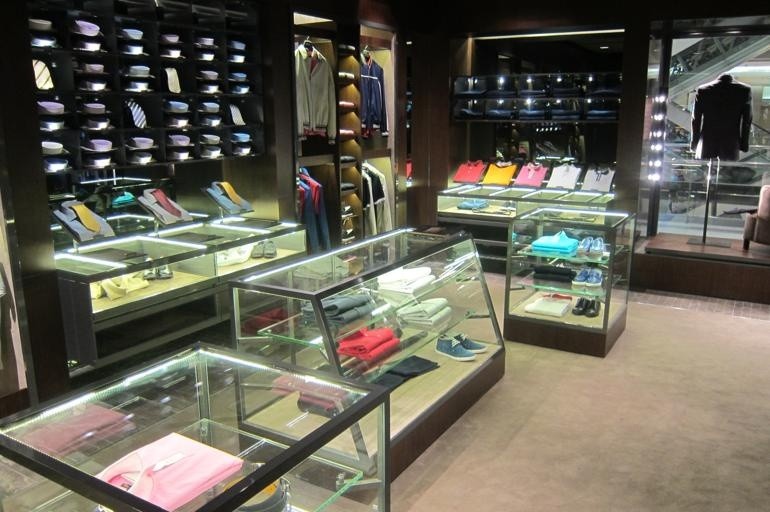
[166,68,182,94]
[151,189,182,218]
[32,59,55,91]
[125,100,147,129]
[230,104,246,127]
[69,205,100,233]
[217,181,242,206]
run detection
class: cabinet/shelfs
[437,186,617,274]
[503,205,639,359]
[0,339,395,512]
[54,212,307,380]
[2,0,277,248]
[277,0,450,257]
[225,225,508,507]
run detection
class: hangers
[298,30,314,50]
[360,42,372,60]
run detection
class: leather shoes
[572,297,601,317]
[578,236,604,256]
[144,256,173,280]
[571,266,603,287]
[251,240,277,259]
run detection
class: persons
[691,72,753,161]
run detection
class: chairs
[741,183,770,252]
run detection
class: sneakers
[455,333,487,354]
[436,334,476,362]
